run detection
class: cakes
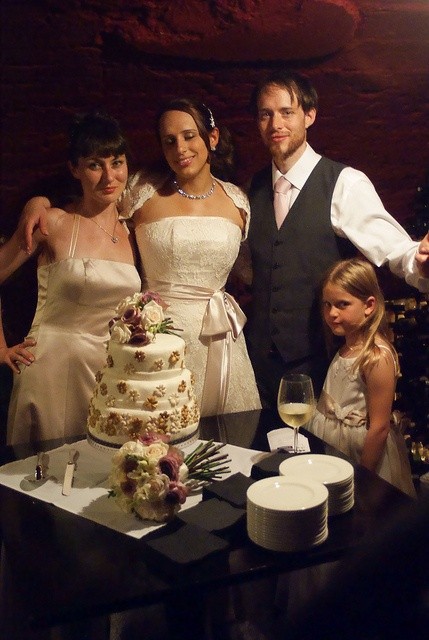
[86,331,201,463]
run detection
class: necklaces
[80,205,119,244]
[171,178,215,200]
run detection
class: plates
[246,475,329,553]
[277,454,355,515]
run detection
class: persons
[244,71,429,451]
[0,118,142,445]
[15,94,263,418]
[304,257,419,502]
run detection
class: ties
[273,176,293,231]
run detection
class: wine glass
[277,374,317,455]
